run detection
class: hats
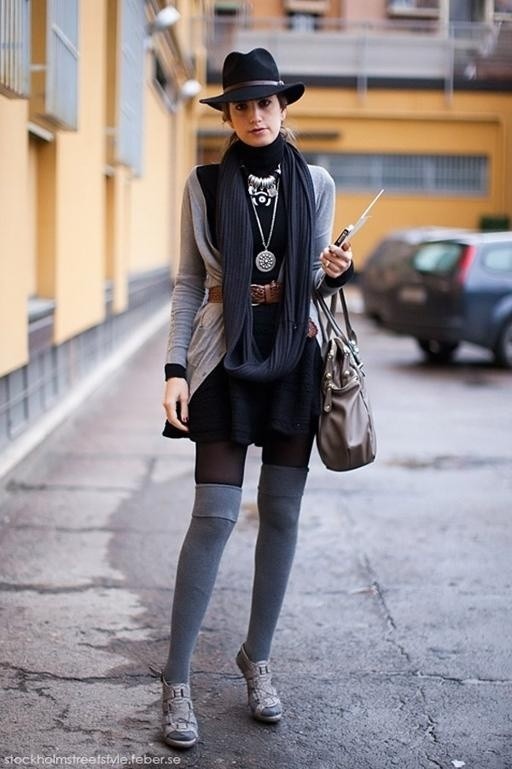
[196,47,305,112]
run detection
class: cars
[358,226,512,369]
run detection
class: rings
[325,260,330,267]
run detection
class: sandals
[148,666,199,749]
[234,642,283,723]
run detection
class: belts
[199,280,284,304]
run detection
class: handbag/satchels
[311,271,378,471]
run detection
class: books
[314,189,385,290]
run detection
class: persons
[160,48,354,749]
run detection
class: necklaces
[239,159,282,207]
[249,177,282,273]
